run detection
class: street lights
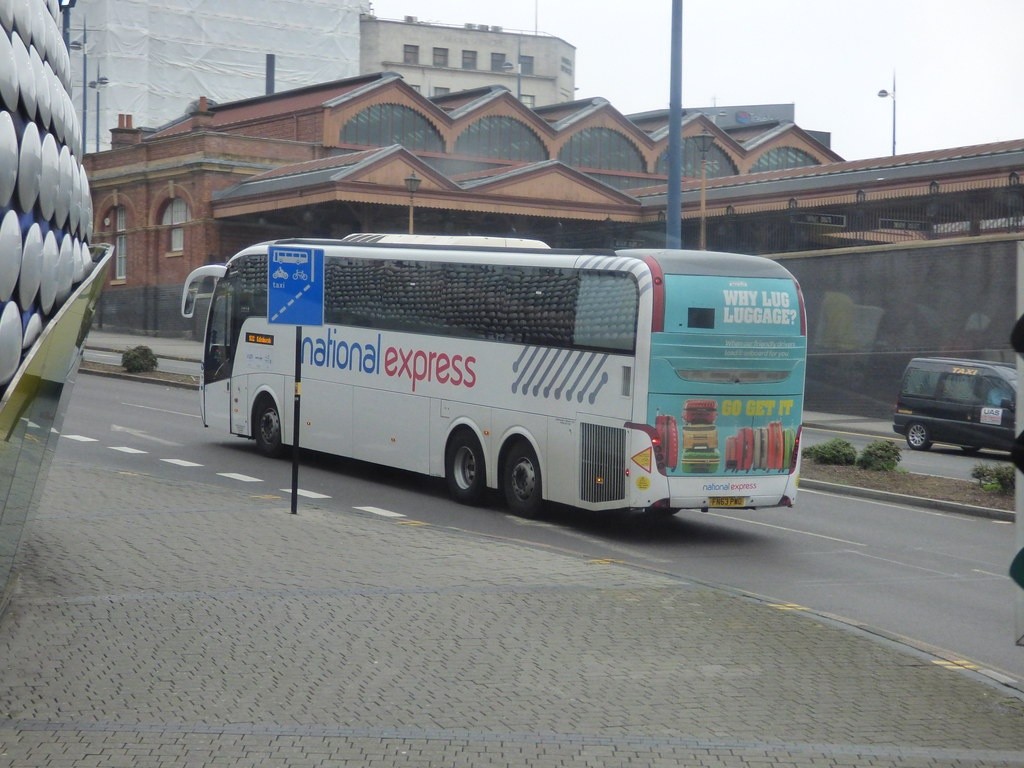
[90,77,109,153]
[71,40,86,156]
[877,89,896,156]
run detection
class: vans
[892,354,1016,453]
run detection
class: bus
[181,233,808,523]
